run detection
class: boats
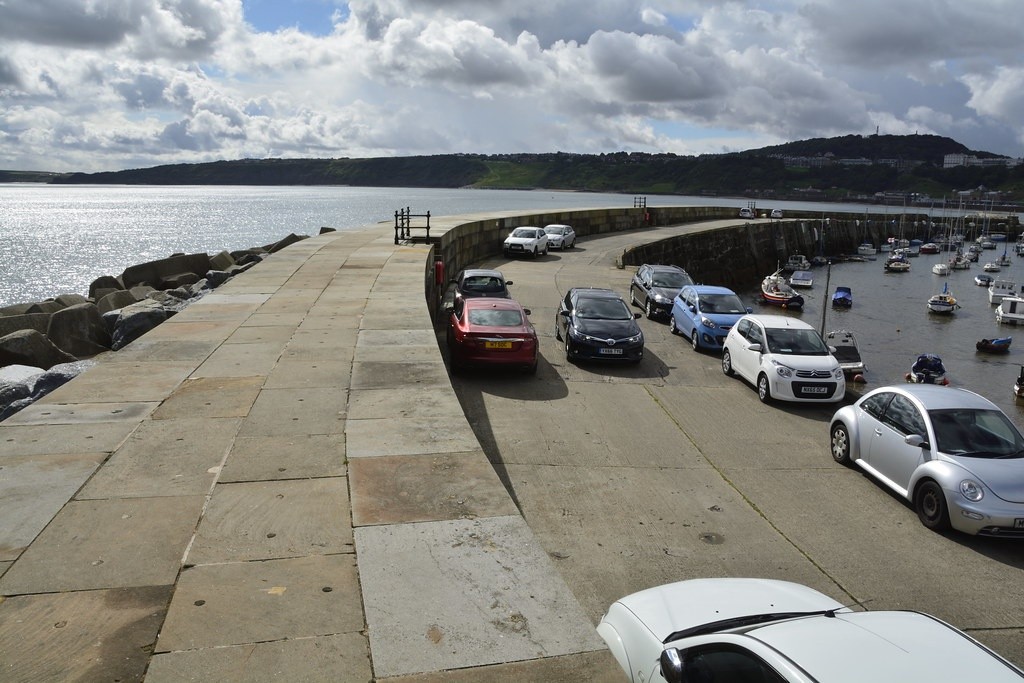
[782,251,811,272]
[975,336,1013,354]
[823,328,867,383]
[761,260,804,309]
[830,284,854,307]
[905,353,950,388]
[790,268,815,289]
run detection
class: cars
[446,298,539,372]
[447,268,513,306]
[554,287,644,365]
[597,574,1024,683]
[740,208,755,219]
[828,383,1023,540]
[503,226,549,258]
[721,314,846,406]
[668,285,756,352]
[542,224,576,249]
[770,208,784,219]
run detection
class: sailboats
[852,194,1024,327]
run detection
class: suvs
[629,263,695,321]
